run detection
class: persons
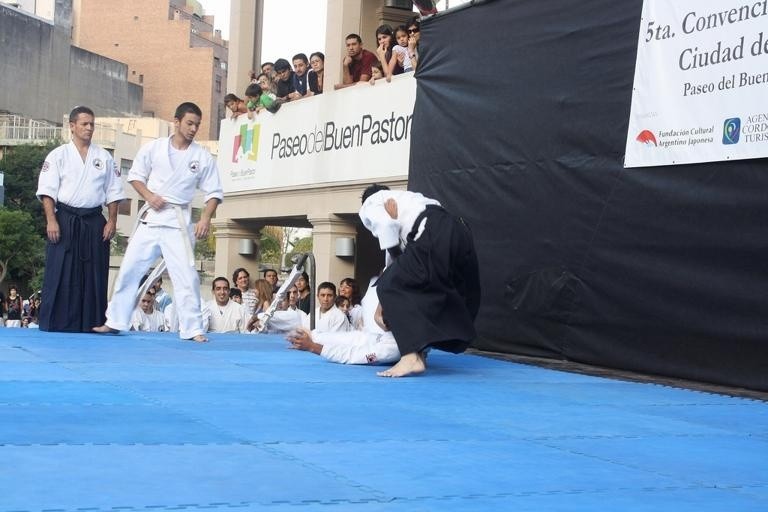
[153,276,172,312]
[291,53,319,100]
[229,288,242,305]
[133,291,166,332]
[359,184,483,377]
[288,283,307,316]
[287,271,312,315]
[369,61,384,85]
[163,299,211,331]
[406,16,421,71]
[28,298,36,317]
[36,106,127,335]
[263,269,281,293]
[22,318,29,328]
[247,287,302,333]
[31,298,40,320]
[262,62,274,81]
[253,278,274,315]
[255,73,277,103]
[150,288,160,311]
[274,58,297,104]
[92,102,224,342]
[284,197,408,365]
[224,94,247,120]
[335,294,351,323]
[386,25,413,83]
[304,282,350,333]
[342,33,384,85]
[376,24,404,77]
[28,316,39,328]
[5,286,23,328]
[245,84,274,120]
[309,52,325,96]
[0,289,5,326]
[232,268,258,314]
[269,70,281,84]
[338,278,361,309]
[207,278,245,333]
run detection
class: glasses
[407,28,419,34]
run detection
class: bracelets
[286,94,289,100]
[409,54,415,59]
